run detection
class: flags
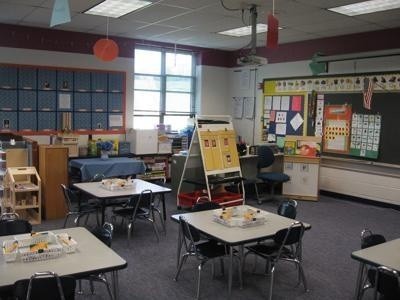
[362,77,373,110]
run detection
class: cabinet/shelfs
[6,167,43,225]
[38,144,69,223]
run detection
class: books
[137,156,168,179]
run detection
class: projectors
[236,55,268,68]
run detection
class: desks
[0,227,127,300]
[172,153,203,185]
[224,153,286,201]
[351,238,400,300]
[69,156,146,181]
[282,154,320,202]
[128,153,170,184]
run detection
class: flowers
[96,141,114,150]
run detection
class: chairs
[0,213,32,236]
[257,144,290,205]
[368,266,400,299]
[360,229,387,289]
[60,174,309,299]
[14,272,76,300]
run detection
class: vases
[101,150,108,159]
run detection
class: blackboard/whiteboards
[306,90,400,168]
